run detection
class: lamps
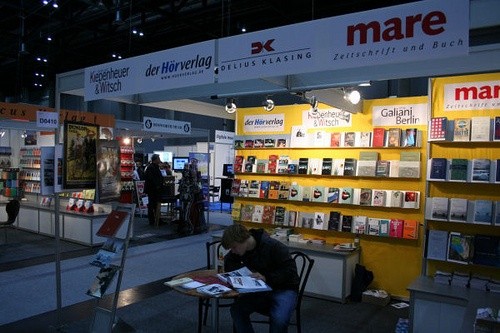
[262,95,275,111]
[289,91,317,108]
[223,97,236,114]
[342,86,361,104]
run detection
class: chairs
[199,241,237,332]
[249,250,314,333]
[0,200,20,242]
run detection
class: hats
[151,154,159,160]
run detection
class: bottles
[354,233,360,248]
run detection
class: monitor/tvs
[172,156,189,170]
[223,164,233,176]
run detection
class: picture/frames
[96,139,122,203]
[62,120,100,190]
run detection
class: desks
[171,269,244,333]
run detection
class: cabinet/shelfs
[423,138,499,269]
[0,201,108,246]
[209,229,362,305]
[231,145,422,240]
[406,273,500,333]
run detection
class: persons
[221,223,300,333]
[144,154,163,225]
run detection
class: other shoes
[152,222,163,226]
[150,223,152,225]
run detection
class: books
[427,230,500,268]
[431,197,500,224]
[96,210,131,240]
[434,269,499,293]
[426,116,500,141]
[0,149,95,214]
[164,266,272,297]
[90,238,125,266]
[428,158,500,181]
[231,126,423,240]
